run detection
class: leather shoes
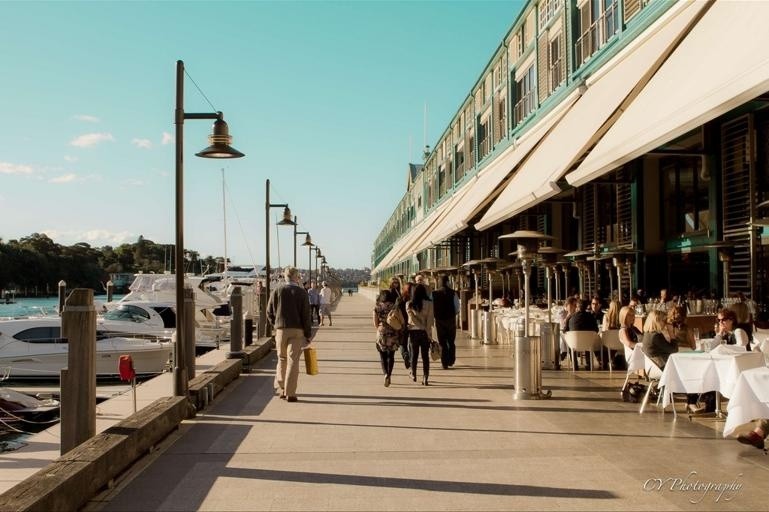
[737,430,764,448]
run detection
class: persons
[318,280,333,326]
[348,288,351,296]
[554,288,769,450]
[350,291,353,296]
[263,265,313,402]
[371,273,461,387]
[254,279,271,311]
[307,279,320,325]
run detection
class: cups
[720,340,727,344]
[721,297,742,305]
[703,340,711,352]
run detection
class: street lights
[309,245,325,291]
[174,58,245,399]
[316,255,335,290]
[262,178,298,338]
[292,214,315,268]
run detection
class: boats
[0,318,175,380]
[0,365,60,432]
[98,302,218,355]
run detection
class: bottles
[704,299,717,315]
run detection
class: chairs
[481,293,769,448]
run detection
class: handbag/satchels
[387,310,402,329]
[304,347,318,375]
[430,340,442,360]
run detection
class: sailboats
[94,168,309,348]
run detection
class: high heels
[685,403,705,414]
